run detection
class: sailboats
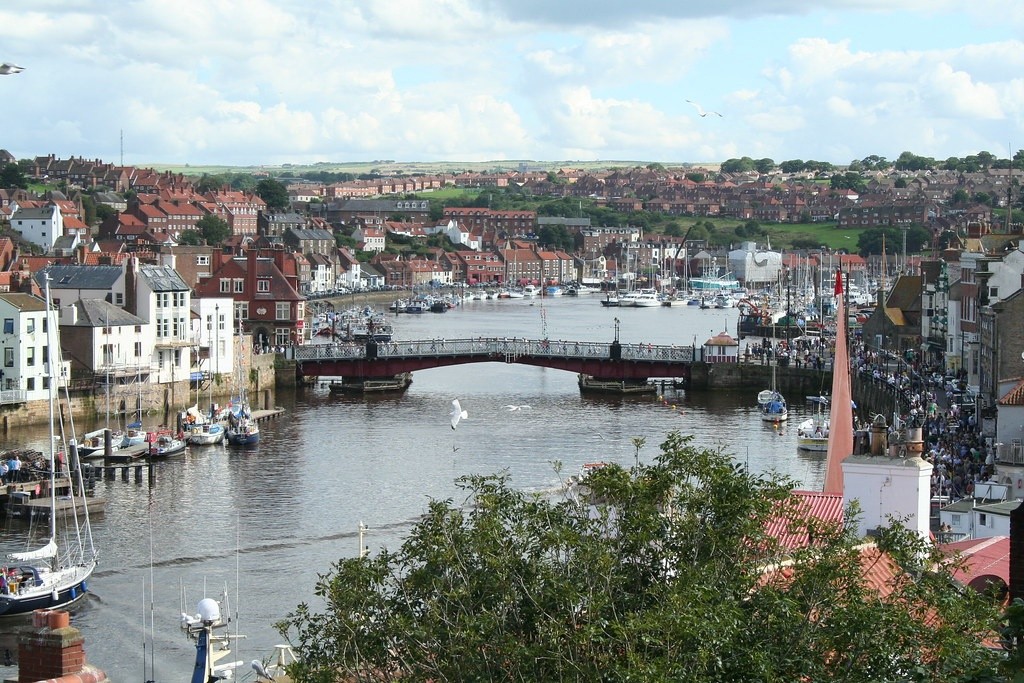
[601,245,891,347]
[0,271,262,616]
[757,322,792,422]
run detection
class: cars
[359,283,405,291]
[305,287,358,299]
[408,280,470,291]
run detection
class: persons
[744,332,993,498]
[253,336,677,358]
[0,566,21,595]
[0,455,41,487]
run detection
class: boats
[797,391,829,452]
[471,280,590,301]
[466,292,474,304]
[391,292,465,314]
[312,304,394,344]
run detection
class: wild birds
[505,404,531,411]
[685,99,723,119]
[447,399,468,431]
